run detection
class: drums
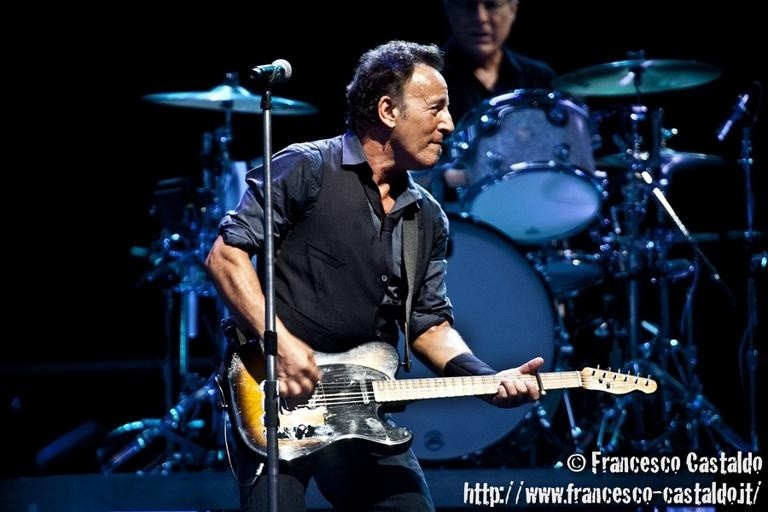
[448,87,606,246]
[395,210,573,462]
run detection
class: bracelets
[443,352,497,401]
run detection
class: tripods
[100,309,235,475]
[558,92,757,472]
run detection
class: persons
[203,43,544,512]
[412,0,554,192]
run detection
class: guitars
[221,339,657,468]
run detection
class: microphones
[254,57,294,84]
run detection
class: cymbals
[146,83,319,117]
[595,145,719,170]
[552,59,725,96]
